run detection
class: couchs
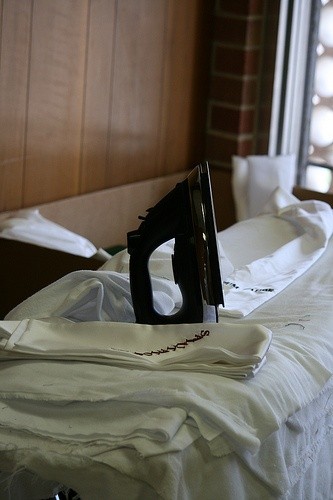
[1,168,333,500]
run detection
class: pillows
[245,155,299,220]
[232,155,251,221]
